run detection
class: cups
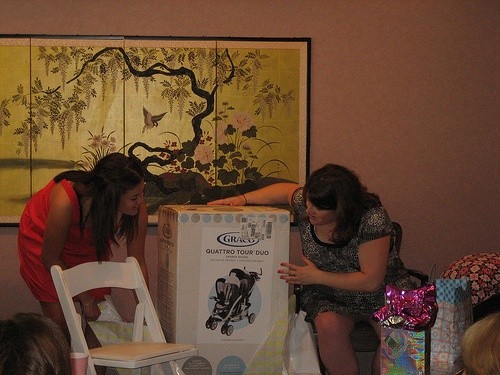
[70,352,89,375]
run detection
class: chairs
[50,256,199,375]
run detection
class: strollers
[205,266,263,337]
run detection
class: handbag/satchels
[428,263,473,375]
[368,317,431,375]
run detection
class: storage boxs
[155,204,290,375]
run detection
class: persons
[0,312,72,375]
[441,253,500,375]
[207,163,415,375]
[17,152,151,375]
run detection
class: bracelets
[242,194,247,205]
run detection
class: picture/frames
[0,33,311,228]
